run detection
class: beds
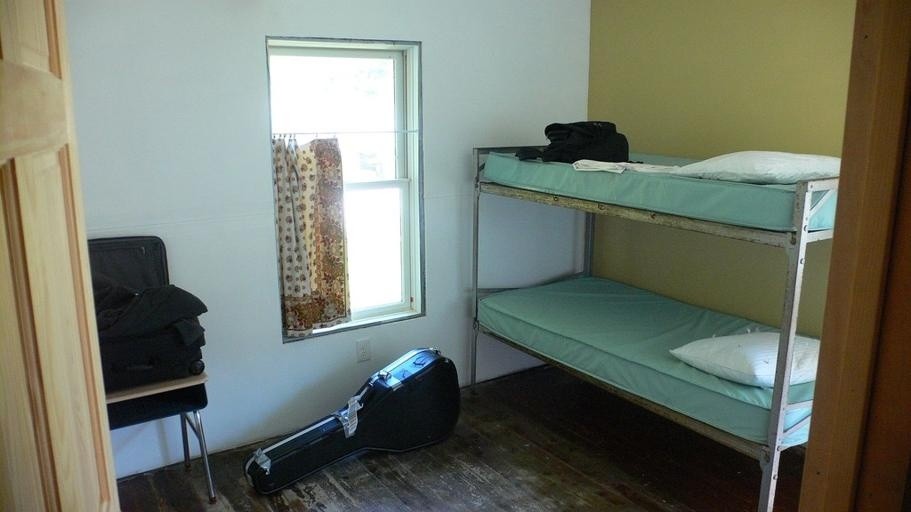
[470,147,842,512]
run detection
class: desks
[105,370,217,504]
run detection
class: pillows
[669,150,842,184]
[669,330,819,388]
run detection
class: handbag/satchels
[542,121,629,164]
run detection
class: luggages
[88,236,208,394]
